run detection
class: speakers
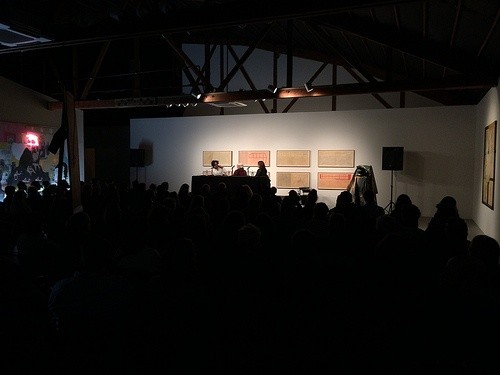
[381,146,404,170]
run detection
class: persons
[234,163,247,176]
[0,177,500,375]
[255,161,267,176]
[209,160,223,174]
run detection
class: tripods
[383,171,396,214]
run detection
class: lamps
[191,91,201,99]
[267,84,278,93]
[304,81,313,92]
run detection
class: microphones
[232,165,235,169]
[247,166,250,170]
[129,148,153,167]
